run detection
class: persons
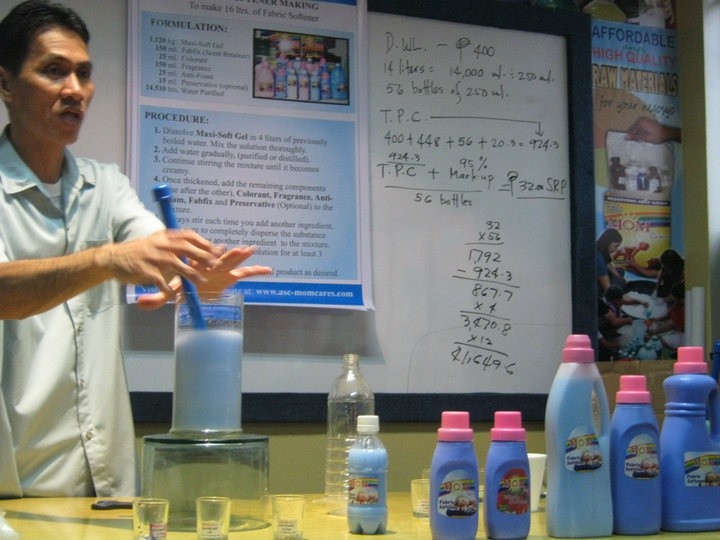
[608,157,625,191]
[0,0,273,500]
[595,229,685,361]
[644,166,662,193]
[624,116,682,145]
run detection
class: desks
[0,490,720,540]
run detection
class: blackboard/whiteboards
[0,0,598,423]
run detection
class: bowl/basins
[140,433,272,531]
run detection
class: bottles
[323,353,374,515]
[544,334,614,538]
[658,346,720,531]
[609,374,662,535]
[609,156,661,194]
[483,411,532,540]
[428,411,480,540]
[347,414,389,534]
[256,52,348,101]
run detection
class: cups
[421,468,430,481]
[194,495,231,540]
[272,493,305,539]
[170,288,244,434]
[131,498,170,540]
[527,452,547,512]
[478,466,485,501]
[410,478,430,518]
